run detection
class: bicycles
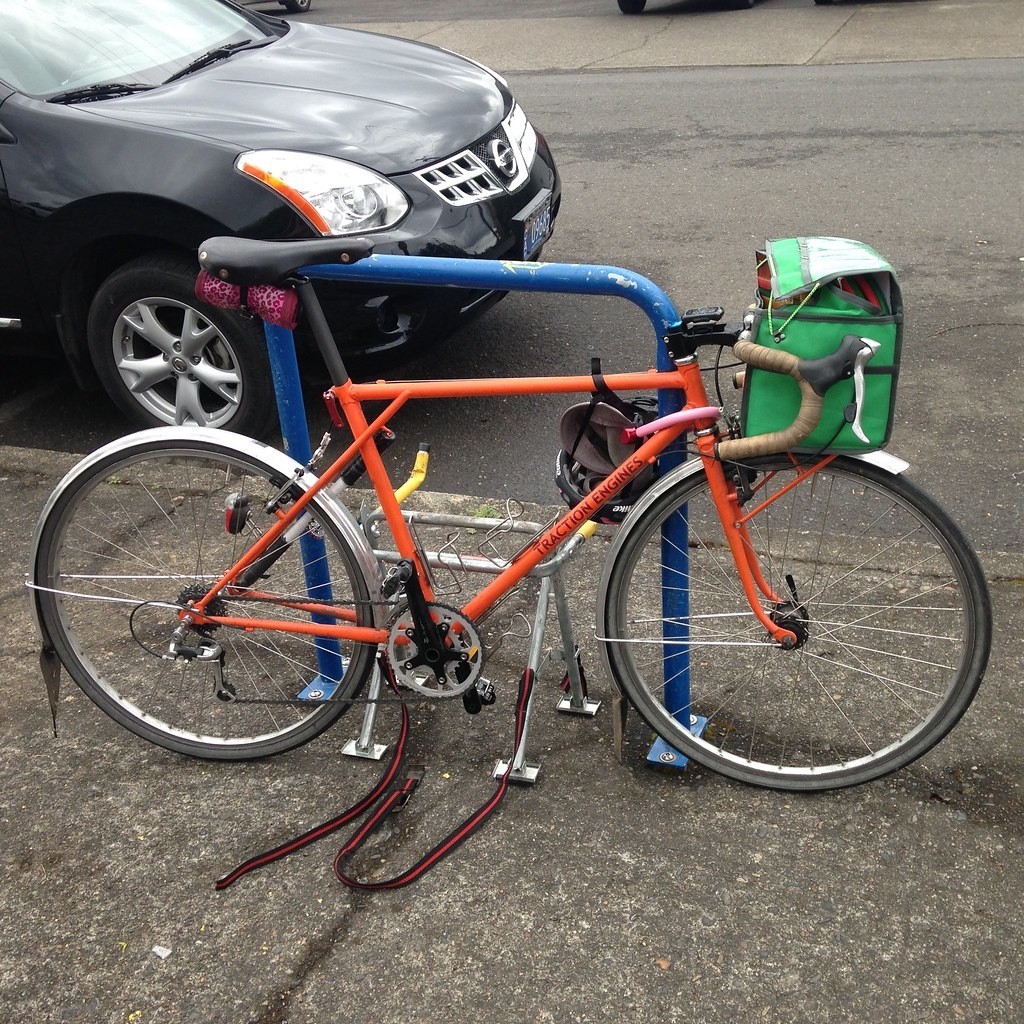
[20,233,996,793]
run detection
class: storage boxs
[735,236,902,455]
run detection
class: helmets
[554,447,664,525]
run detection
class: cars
[0,0,565,438]
